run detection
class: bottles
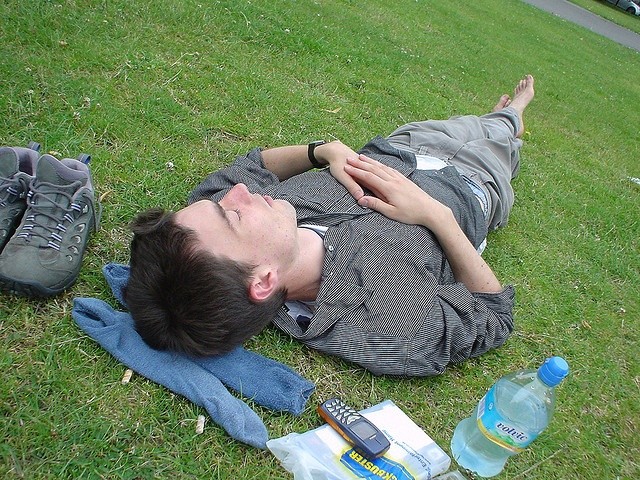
[451,356,569,477]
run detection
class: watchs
[308,139,327,168]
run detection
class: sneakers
[0,140,39,251]
[0,153,102,298]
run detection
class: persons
[122,74,535,378]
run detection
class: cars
[609,0,640,16]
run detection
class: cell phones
[318,395,394,461]
